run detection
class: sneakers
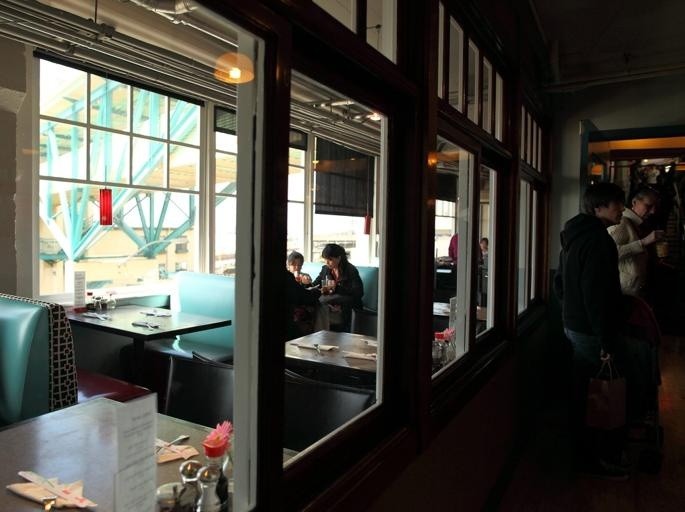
[599,467,628,482]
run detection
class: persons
[629,159,677,195]
[480,237,488,258]
[301,243,364,332]
[287,250,312,285]
[286,267,321,336]
[549,182,631,481]
[606,184,668,299]
[448,232,485,305]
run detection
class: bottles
[155,439,231,512]
[434,331,448,364]
[85,291,116,312]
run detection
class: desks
[2,395,300,512]
[433,301,487,321]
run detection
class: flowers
[443,328,456,351]
[206,420,234,467]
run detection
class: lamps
[214,52,256,84]
[0,0,115,56]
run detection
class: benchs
[0,261,381,428]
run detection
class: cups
[320,275,333,293]
[293,270,302,283]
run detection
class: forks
[154,435,190,454]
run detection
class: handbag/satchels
[587,360,627,429]
